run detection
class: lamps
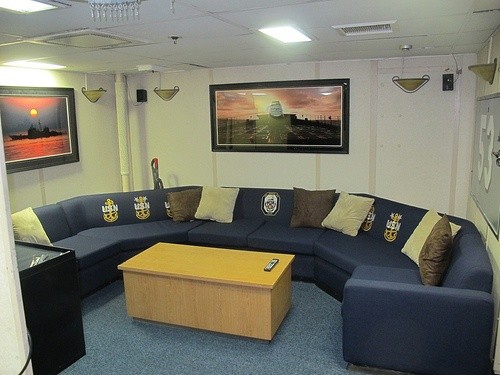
[468,57,497,84]
[154,86,179,101]
[392,75,430,93]
[82,86,107,102]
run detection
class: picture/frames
[209,77,351,154]
[0,85,80,174]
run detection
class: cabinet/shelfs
[15,236,87,375]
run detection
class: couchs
[11,188,494,375]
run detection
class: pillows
[195,185,240,223]
[167,188,202,222]
[401,208,462,268]
[321,190,375,236]
[11,207,53,246]
[290,187,336,228]
[419,212,452,287]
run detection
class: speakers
[137,89,147,102]
[443,74,453,91]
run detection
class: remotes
[264,259,279,271]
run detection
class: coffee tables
[117,242,295,341]
